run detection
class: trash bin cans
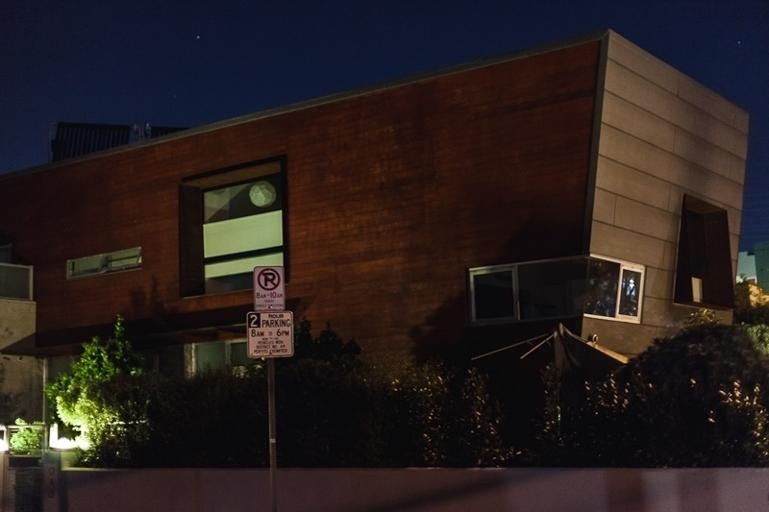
[3,455,43,512]
